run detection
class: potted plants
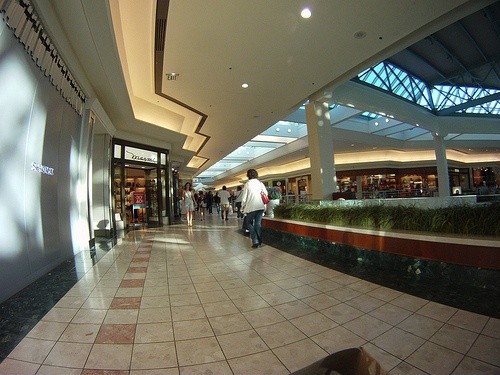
[266,187,280,216]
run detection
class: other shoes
[189,222,193,227]
[187,222,189,226]
[225,217,229,220]
[237,214,240,218]
[201,218,203,221]
[252,242,261,248]
[221,217,224,219]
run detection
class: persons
[288,190,293,199]
[281,189,285,200]
[241,169,268,250]
[234,186,243,218]
[214,192,221,213]
[182,182,198,226]
[218,185,230,220]
[205,191,213,214]
[193,191,208,221]
[129,186,140,223]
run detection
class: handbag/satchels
[202,202,207,208]
[261,192,270,204]
